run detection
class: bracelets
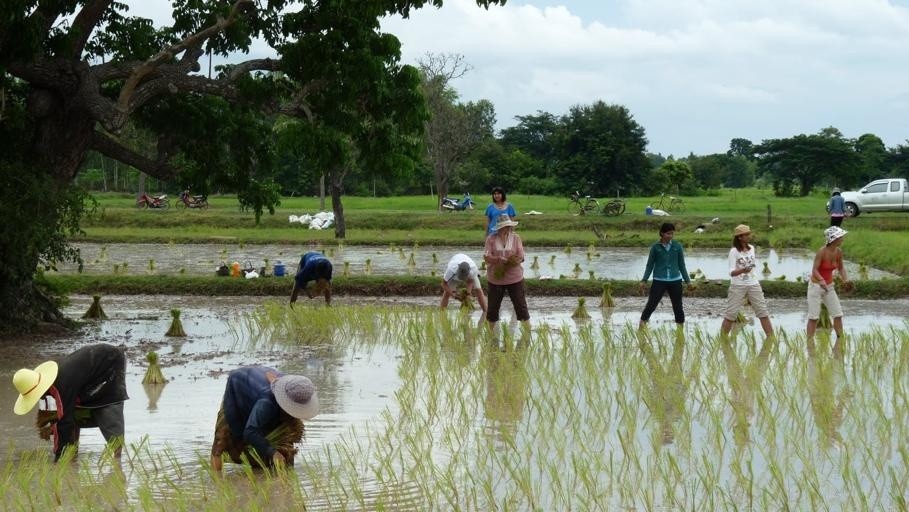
[817,278,824,281]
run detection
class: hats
[315,258,332,277]
[12,360,58,417]
[456,262,471,280]
[270,374,321,420]
[832,187,841,194]
[490,213,519,232]
[733,224,752,237]
[822,225,849,245]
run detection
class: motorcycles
[137,192,170,210]
[441,193,476,210]
[176,188,208,209]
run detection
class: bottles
[233,260,240,277]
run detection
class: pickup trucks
[826,178,909,217]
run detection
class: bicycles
[651,193,685,214]
[567,191,599,217]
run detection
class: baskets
[215,260,230,276]
[240,260,256,278]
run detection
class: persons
[806,226,849,337]
[12,343,129,463]
[211,365,320,471]
[720,224,776,337]
[440,253,487,312]
[640,224,692,329]
[484,186,515,242]
[484,213,532,332]
[289,252,333,309]
[827,187,847,227]
